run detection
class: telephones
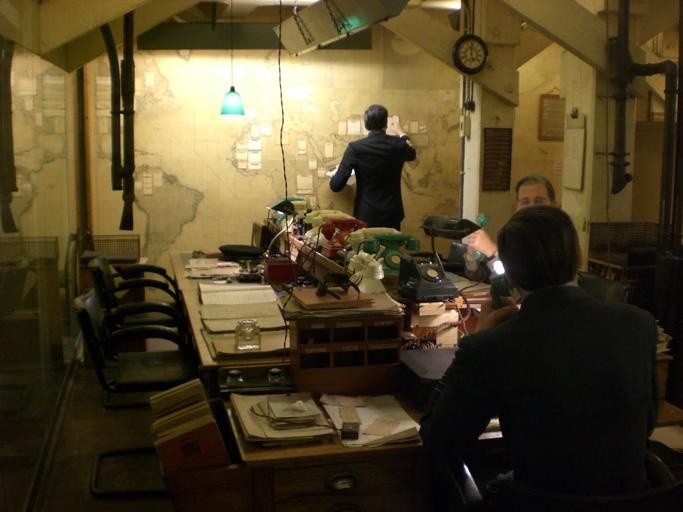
[399,257,458,302]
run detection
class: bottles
[296,275,312,289]
[233,320,261,352]
[291,208,312,240]
[267,368,284,382]
[489,275,513,310]
[225,369,242,384]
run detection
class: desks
[171,248,682,510]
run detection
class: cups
[238,257,263,274]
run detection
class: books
[147,280,417,478]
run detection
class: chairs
[85,254,181,410]
[72,286,193,497]
[414,416,683,512]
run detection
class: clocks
[452,33,489,76]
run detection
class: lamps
[422,215,482,254]
[219,0,248,119]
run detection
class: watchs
[486,245,499,260]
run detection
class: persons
[418,205,661,512]
[329,102,416,231]
[512,172,556,211]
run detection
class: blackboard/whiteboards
[483,128,512,192]
